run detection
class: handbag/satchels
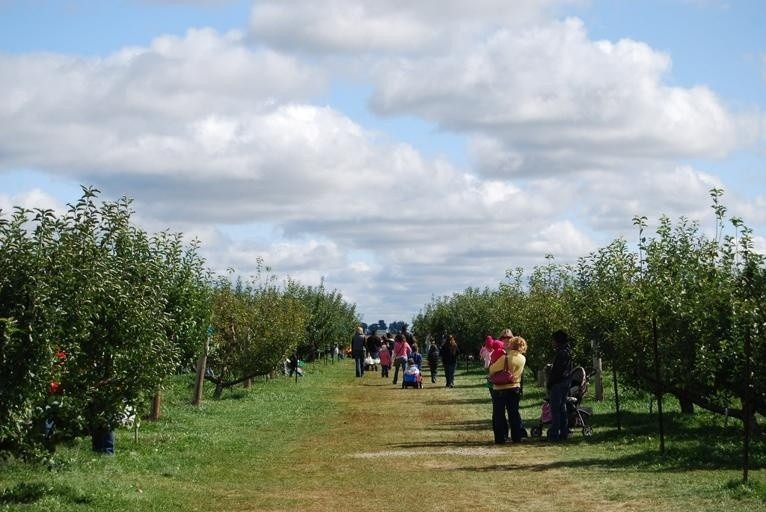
[489,355,515,385]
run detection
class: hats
[551,328,568,344]
[485,336,494,347]
[497,329,514,339]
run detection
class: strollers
[529,366,594,439]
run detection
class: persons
[545,329,573,442]
[490,339,505,362]
[479,335,495,371]
[332,323,462,389]
[490,335,529,444]
[487,329,515,402]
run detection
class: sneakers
[372,365,455,388]
